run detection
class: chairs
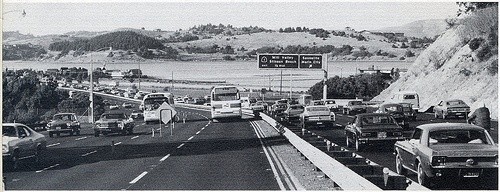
[455,132,471,144]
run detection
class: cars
[93,112,135,138]
[47,112,81,137]
[343,113,406,152]
[1,122,47,172]
[393,122,498,190]
[244,91,420,123]
[432,99,471,120]
[40,77,212,117]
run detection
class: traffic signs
[298,53,324,70]
[256,52,299,70]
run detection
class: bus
[143,92,175,125]
[210,85,243,121]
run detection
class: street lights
[0,0,27,189]
